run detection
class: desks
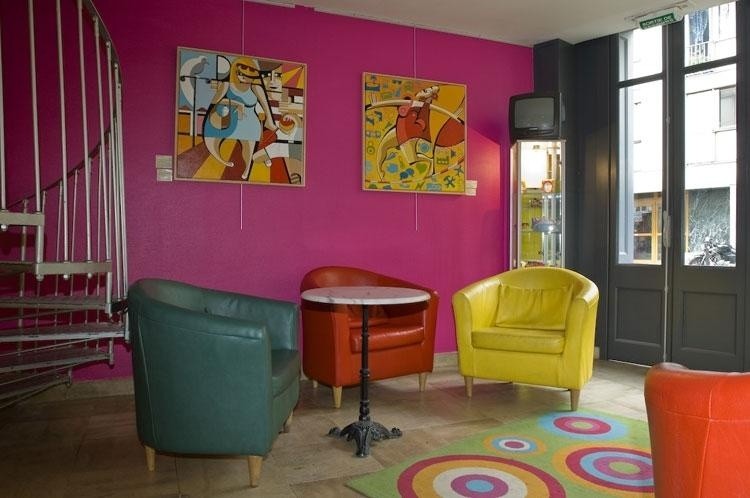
[300,285,432,460]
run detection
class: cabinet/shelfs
[509,137,567,268]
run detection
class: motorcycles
[690,238,736,264]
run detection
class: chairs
[643,360,750,498]
[127,277,303,489]
[298,266,442,406]
[451,267,600,411]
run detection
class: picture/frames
[174,46,309,188]
[361,69,468,196]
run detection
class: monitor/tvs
[508,91,566,144]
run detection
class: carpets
[343,407,656,498]
